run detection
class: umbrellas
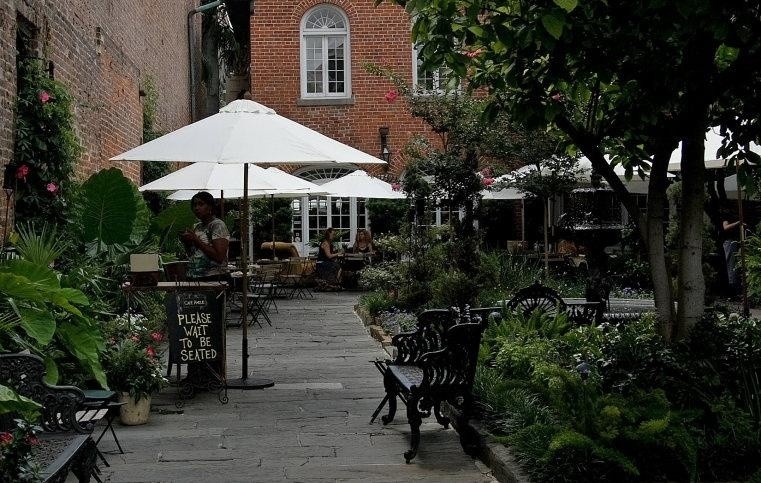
[165,189,288,256]
[108,88,390,380]
[264,165,330,256]
[470,153,586,277]
[575,152,677,195]
[313,168,412,228]
[668,106,761,318]
[137,160,312,221]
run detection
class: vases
[118,390,152,426]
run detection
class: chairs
[232,255,319,328]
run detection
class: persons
[316,227,347,291]
[353,228,374,264]
[176,191,235,303]
[718,197,745,299]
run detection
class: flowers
[99,335,170,405]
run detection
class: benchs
[1,352,98,482]
[381,306,483,464]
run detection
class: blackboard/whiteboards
[168,290,225,364]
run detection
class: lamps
[380,126,393,172]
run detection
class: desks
[157,279,231,408]
[369,356,417,424]
[45,408,109,475]
